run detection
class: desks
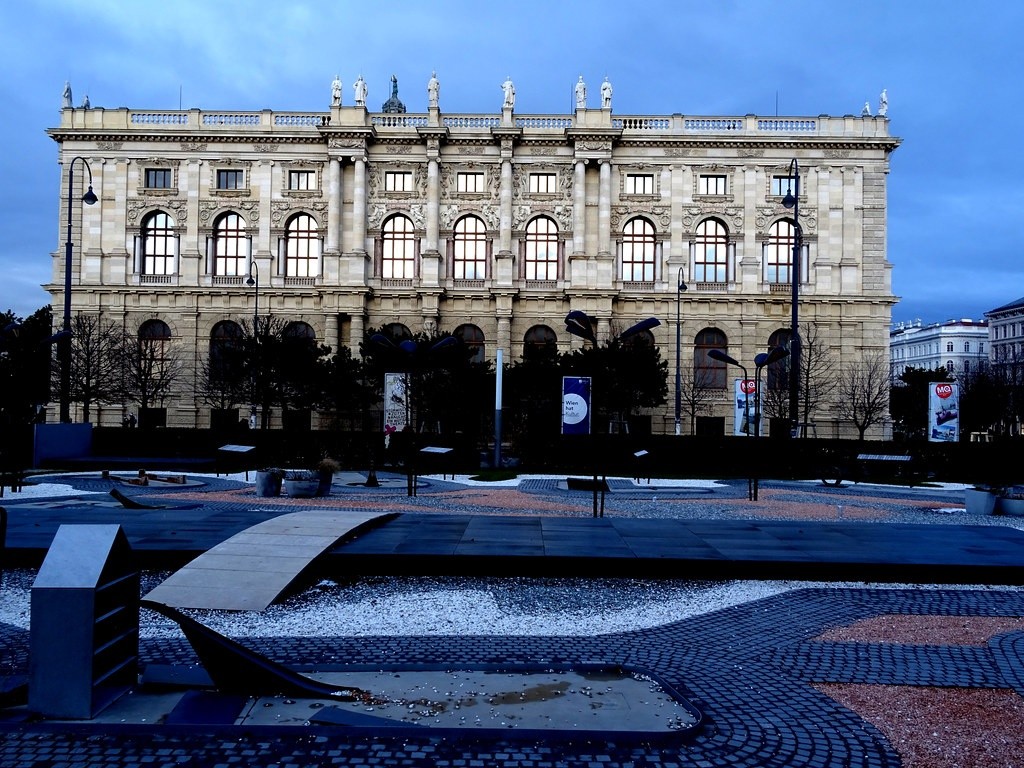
[217,444,256,481]
[420,446,454,480]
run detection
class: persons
[353,74,368,106]
[83,95,90,109]
[501,75,515,107]
[601,77,613,107]
[575,76,587,108]
[330,73,342,106]
[63,81,72,107]
[877,89,888,117]
[860,102,871,116]
[428,71,440,107]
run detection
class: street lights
[247,260,261,454]
[371,328,457,499]
[779,157,802,434]
[564,310,661,517]
[674,266,689,433]
[706,344,790,501]
[60,155,100,424]
